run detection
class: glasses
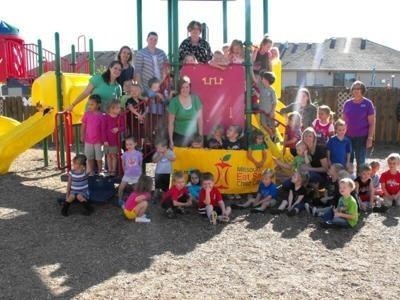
[352,87,361,90]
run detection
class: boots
[61,200,71,217]
[80,201,94,216]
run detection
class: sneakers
[304,202,313,216]
[269,207,281,215]
[312,206,319,217]
[135,216,151,223]
[209,211,218,225]
[164,210,178,221]
[250,208,264,214]
[218,216,230,224]
[285,209,296,218]
[175,207,185,215]
[141,213,147,217]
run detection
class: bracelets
[70,103,76,107]
[368,136,374,139]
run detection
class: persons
[271,142,311,172]
[209,34,279,70]
[160,171,193,218]
[122,79,148,110]
[280,88,317,129]
[253,72,278,141]
[184,55,197,64]
[303,203,336,218]
[168,76,204,148]
[301,128,329,182]
[327,120,351,170]
[189,124,267,150]
[271,173,306,215]
[235,171,278,212]
[380,154,400,206]
[186,170,202,200]
[324,178,358,226]
[117,136,143,205]
[117,46,134,85]
[126,84,148,138]
[319,164,343,203]
[102,99,124,176]
[371,162,383,195]
[355,165,374,208]
[124,176,153,224]
[80,94,104,177]
[197,173,231,225]
[342,81,375,168]
[64,60,121,112]
[135,33,168,88]
[61,154,93,216]
[151,139,176,196]
[150,77,166,133]
[313,105,335,140]
[284,114,302,157]
[180,21,213,62]
